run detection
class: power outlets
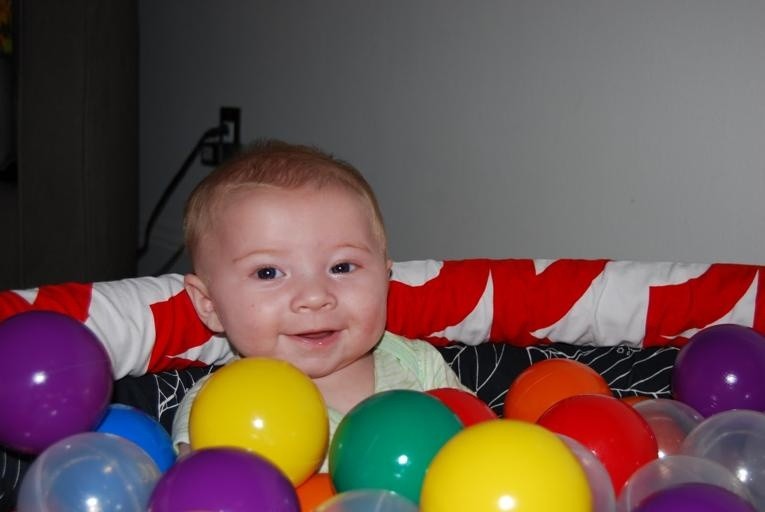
[216,107,240,160]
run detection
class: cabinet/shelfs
[0,1,141,291]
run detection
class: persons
[171,139,476,474]
